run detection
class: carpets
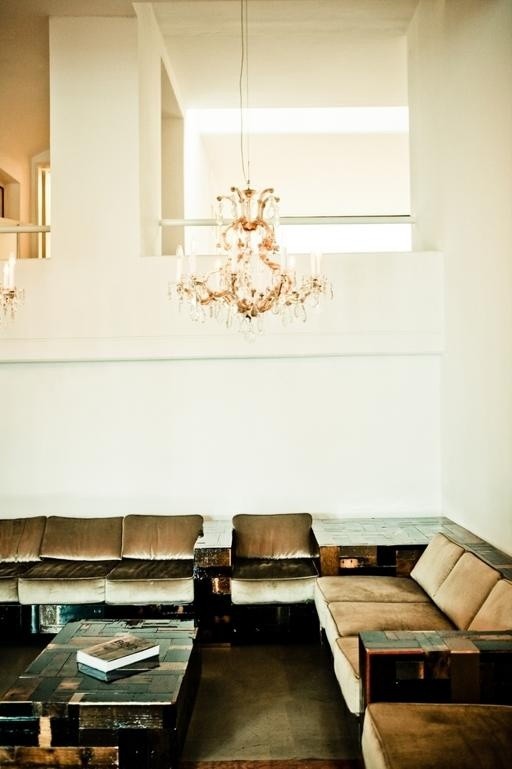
[0,632,363,769]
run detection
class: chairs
[229,514,322,644]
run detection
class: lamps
[172,1,330,333]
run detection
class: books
[76,633,161,674]
[75,654,161,685]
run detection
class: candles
[1,251,21,315]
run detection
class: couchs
[1,515,204,634]
